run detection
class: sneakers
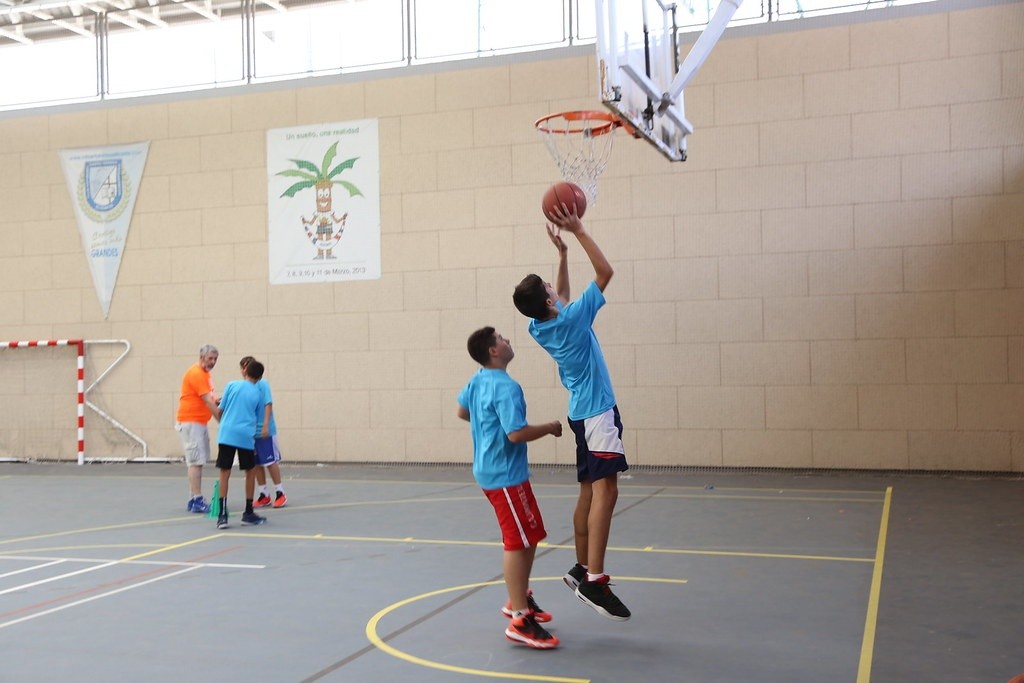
[187,496,209,513]
[216,515,228,529]
[252,492,271,508]
[574,574,631,621]
[505,611,560,650]
[563,564,589,590]
[501,589,552,623]
[272,490,287,508]
[241,512,267,525]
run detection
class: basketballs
[541,180,587,225]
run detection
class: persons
[513,202,631,621]
[240,357,287,508]
[457,327,562,648]
[174,344,220,513]
[216,362,267,528]
